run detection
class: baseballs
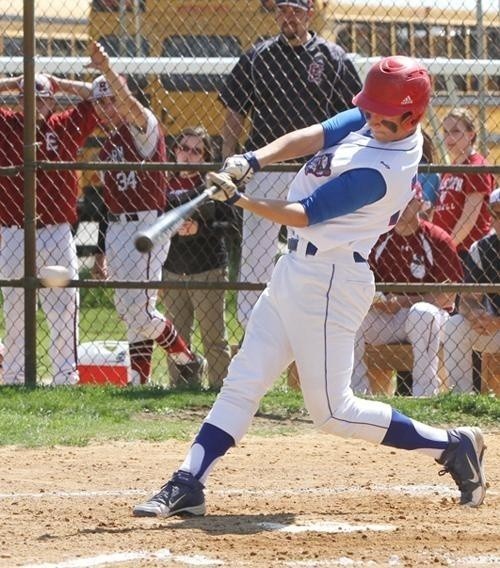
[39,265,71,287]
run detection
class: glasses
[178,142,203,155]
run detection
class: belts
[286,236,369,263]
[104,211,138,225]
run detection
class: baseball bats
[135,184,220,253]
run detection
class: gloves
[216,148,261,185]
[203,168,244,206]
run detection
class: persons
[227,0,358,389]
[167,126,235,384]
[0,71,99,384]
[367,184,473,398]
[82,38,207,387]
[133,51,487,516]
[431,115,496,243]
[462,189,500,402]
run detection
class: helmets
[351,53,436,131]
[86,72,129,101]
[17,72,53,98]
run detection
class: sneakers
[434,424,489,510]
[129,468,208,518]
[174,349,210,392]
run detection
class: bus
[80,1,500,227]
